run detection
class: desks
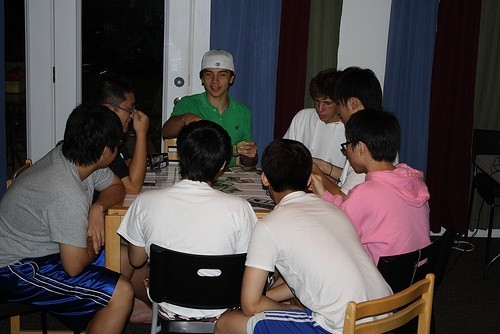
[4,91,26,175]
[103,163,322,275]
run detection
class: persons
[116,120,263,334]
[302,65,402,202]
[162,49,259,169]
[70,69,161,325]
[0,98,136,334]
[281,68,348,186]
[264,107,432,310]
[212,139,395,334]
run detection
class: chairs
[461,128,500,280]
[6,158,88,334]
[149,242,248,334]
[375,228,459,333]
[343,272,435,333]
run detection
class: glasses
[341,140,355,153]
[106,103,140,115]
[116,140,124,147]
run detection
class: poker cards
[232,139,248,157]
[212,165,256,194]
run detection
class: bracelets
[328,162,334,177]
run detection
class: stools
[0,301,49,334]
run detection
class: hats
[201,50,235,72]
